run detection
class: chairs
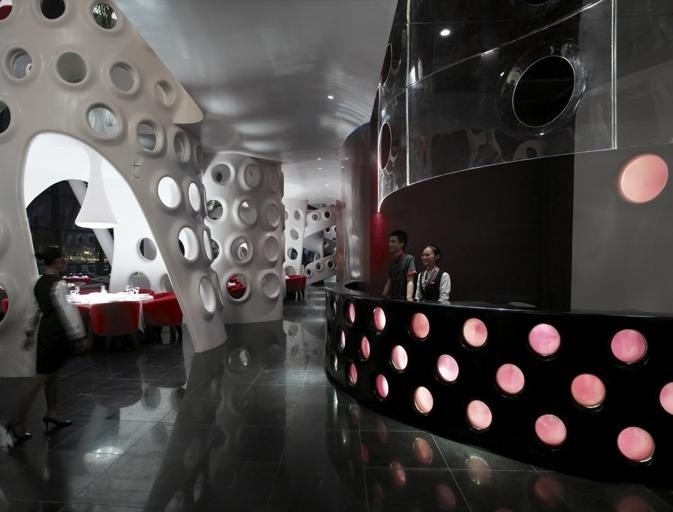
[285,273,307,301]
[67,287,182,374]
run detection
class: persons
[414,245,451,302]
[382,230,417,302]
[7,245,90,441]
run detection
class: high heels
[42,415,72,432]
[5,421,32,441]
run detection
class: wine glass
[134,287,140,294]
